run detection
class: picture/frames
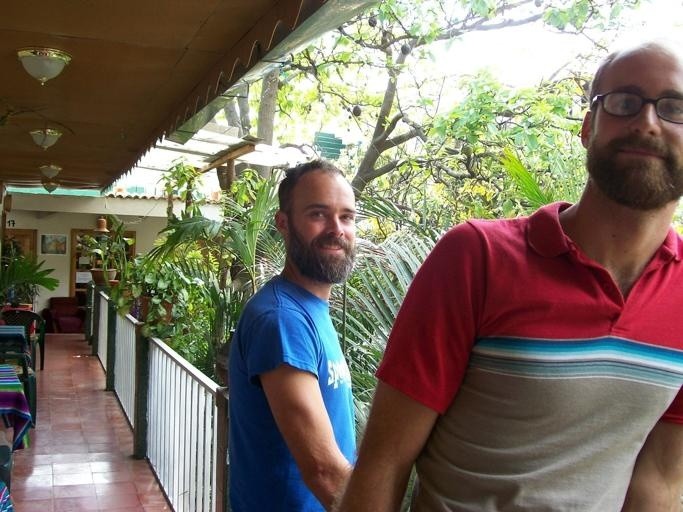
[39,234,68,256]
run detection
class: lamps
[16,46,73,87]
[29,129,62,193]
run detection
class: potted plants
[74,167,284,387]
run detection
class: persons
[227,158,357,512]
[335,41,683,512]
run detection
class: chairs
[42,297,89,334]
[0,311,46,371]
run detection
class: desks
[0,325,33,494]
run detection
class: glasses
[590,90,683,125]
